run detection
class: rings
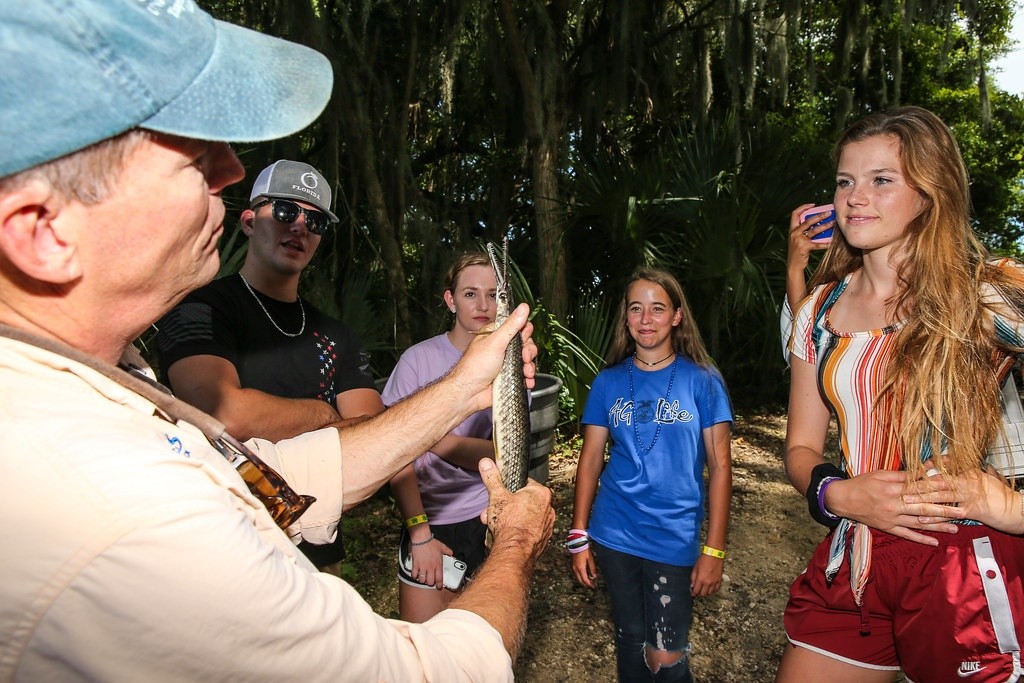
[418,574,426,577]
[804,231,810,238]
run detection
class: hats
[0,0,333,178]
[249,159,340,223]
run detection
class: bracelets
[411,533,434,545]
[702,546,725,558]
[567,529,590,553]
[406,514,430,528]
[807,463,848,528]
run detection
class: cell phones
[404,552,467,592]
[801,204,837,244]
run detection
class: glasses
[251,198,332,235]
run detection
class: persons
[566,269,734,683]
[777,107,1024,683]
[157,160,384,577]
[781,202,835,366]
[0,0,555,683]
[381,252,532,623]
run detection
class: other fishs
[482,236,530,564]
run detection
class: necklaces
[630,352,678,450]
[239,272,305,336]
